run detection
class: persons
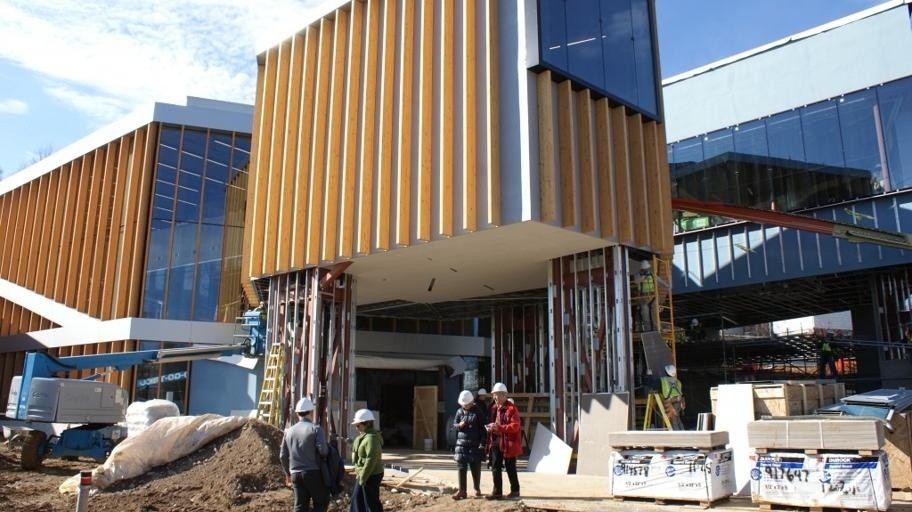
[644,363,686,431]
[632,259,670,332]
[452,389,487,501]
[477,386,494,461]
[349,409,384,512]
[279,396,332,512]
[254,301,268,320]
[485,380,523,499]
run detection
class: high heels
[452,490,467,500]
[473,489,481,497]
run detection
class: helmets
[639,260,651,270]
[457,390,474,406]
[691,319,699,327]
[350,409,376,425]
[491,383,508,394]
[477,388,488,395]
[664,365,677,377]
[827,329,835,334]
[294,397,314,413]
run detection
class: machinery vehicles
[1,311,267,470]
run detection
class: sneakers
[485,493,502,500]
[507,491,520,498]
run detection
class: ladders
[653,255,676,363]
[256,343,285,428]
[643,390,686,430]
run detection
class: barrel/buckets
[424,436,433,454]
